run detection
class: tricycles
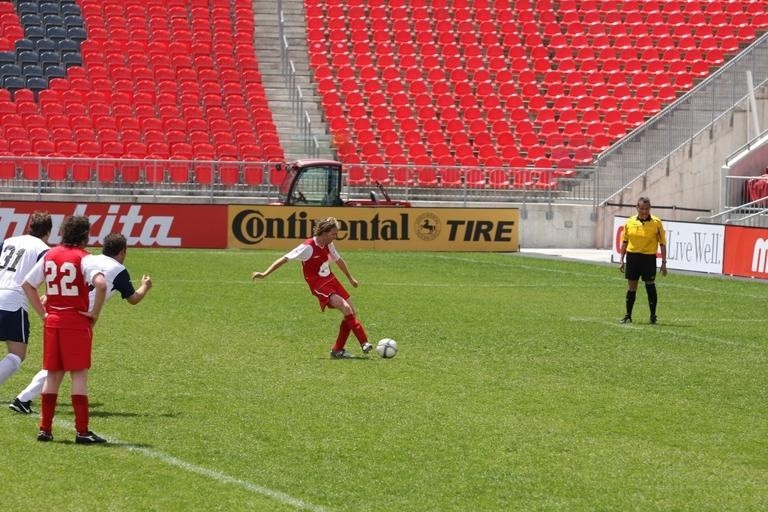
[267,159,411,208]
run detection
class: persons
[252,216,373,358]
[0,210,53,387]
[618,196,668,324]
[21,214,108,444]
[9,233,153,416]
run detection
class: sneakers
[36,430,53,441]
[620,314,632,323]
[360,342,373,353]
[329,350,355,359]
[75,431,107,443]
[9,397,39,416]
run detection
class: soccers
[376,338,398,358]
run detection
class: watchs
[662,260,666,264]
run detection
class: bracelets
[620,259,623,261]
